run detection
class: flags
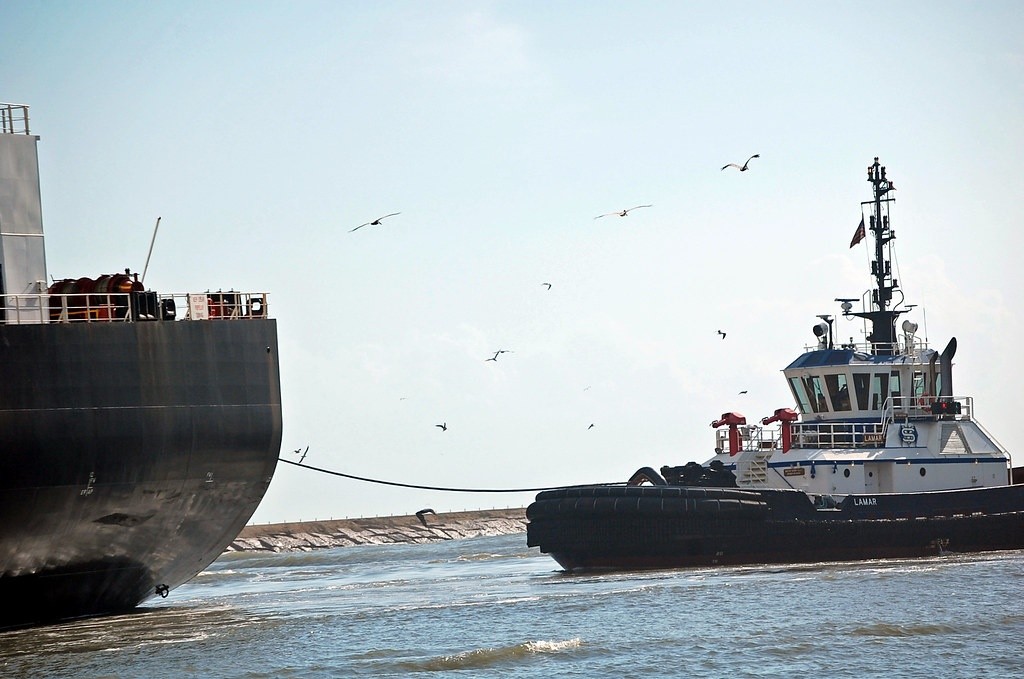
[848,218,868,248]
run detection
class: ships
[0,97,284,630]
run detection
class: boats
[524,151,1024,575]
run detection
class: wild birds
[347,212,404,231]
[588,424,593,429]
[294,445,311,465]
[716,329,729,341]
[739,391,747,395]
[594,205,654,219]
[543,281,553,291]
[722,155,762,171]
[436,422,447,433]
[485,349,513,362]
